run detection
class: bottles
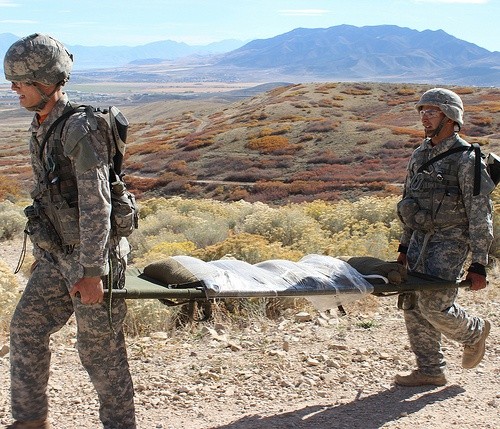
[111,180,129,200]
[25,206,42,233]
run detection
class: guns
[487,152,500,186]
[105,104,130,180]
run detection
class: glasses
[14,81,31,86]
[419,109,442,118]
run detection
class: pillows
[337,255,408,285]
[141,259,203,289]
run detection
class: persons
[396,86,495,384]
[5,33,138,428]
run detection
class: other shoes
[5,420,48,429]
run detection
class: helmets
[415,88,464,125]
[3,33,74,86]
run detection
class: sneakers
[462,319,490,369]
[395,370,447,386]
